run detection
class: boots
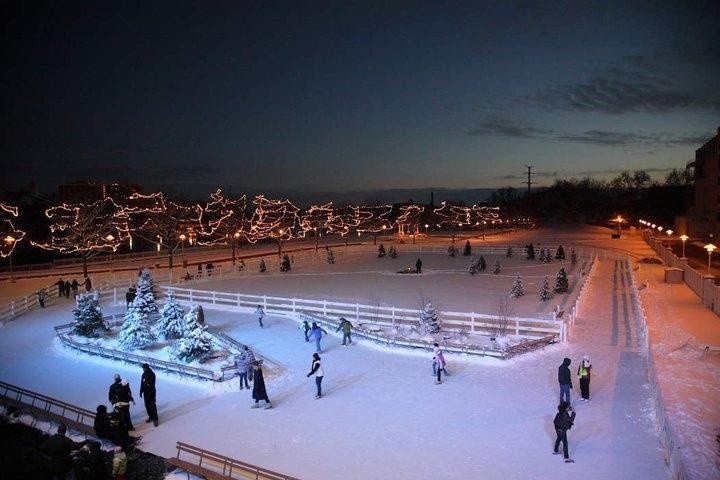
[250,403,260,408]
[263,403,273,409]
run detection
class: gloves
[569,384,573,389]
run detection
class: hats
[114,445,123,453]
[142,363,149,369]
[582,352,590,362]
[114,373,120,380]
[122,379,131,386]
[312,352,319,359]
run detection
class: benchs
[167,441,301,480]
[0,380,107,442]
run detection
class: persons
[435,350,448,384]
[325,242,330,251]
[125,287,135,310]
[109,373,135,431]
[297,320,311,343]
[41,424,128,480]
[335,317,354,346]
[236,345,255,389]
[415,257,423,274]
[182,254,187,268]
[306,353,323,400]
[196,263,203,277]
[552,402,576,463]
[55,278,65,297]
[577,355,592,402]
[80,278,92,291]
[35,289,51,308]
[254,305,266,327]
[557,357,573,409]
[430,343,448,376]
[307,321,327,352]
[64,280,72,298]
[206,262,214,278]
[279,256,282,270]
[291,255,295,263]
[251,361,272,409]
[140,363,159,427]
[238,260,245,272]
[132,286,137,296]
[70,279,80,293]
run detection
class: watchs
[93,404,142,450]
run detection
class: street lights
[657,226,663,238]
[4,235,16,280]
[233,232,241,259]
[665,229,675,250]
[278,229,285,252]
[105,234,116,272]
[704,243,717,274]
[311,216,534,253]
[679,235,691,258]
[179,235,187,255]
[639,219,656,233]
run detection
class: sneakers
[312,393,322,400]
[551,450,561,455]
[145,416,159,428]
[580,397,589,403]
[562,458,575,464]
[340,341,354,346]
[430,372,450,385]
